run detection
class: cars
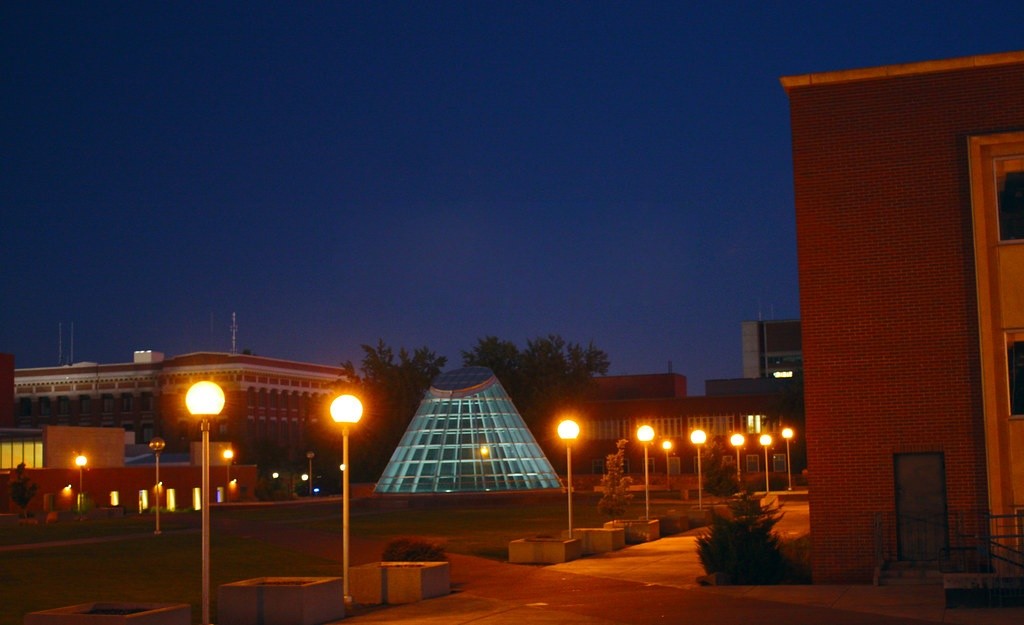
[295,474,340,496]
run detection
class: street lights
[330,394,362,616]
[782,428,795,491]
[760,435,772,492]
[76,456,87,522]
[730,433,745,483]
[636,425,655,521]
[557,420,580,538]
[223,449,233,502]
[186,381,225,625]
[306,451,315,497]
[662,441,672,492]
[691,430,706,511]
[149,437,166,537]
